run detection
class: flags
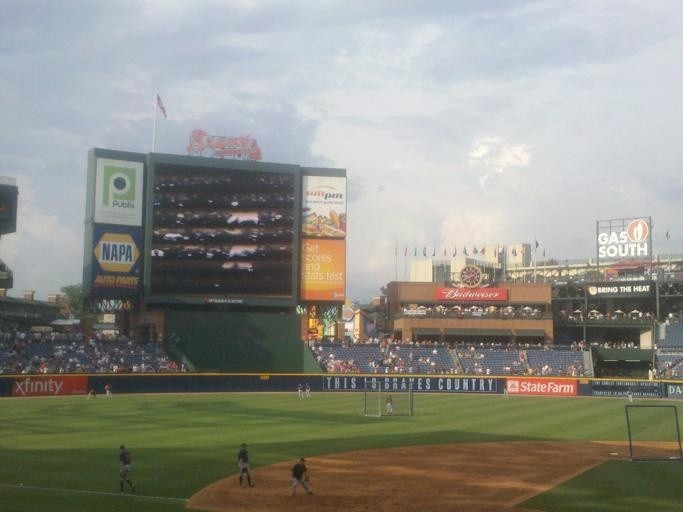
[156,93,167,117]
[394,239,546,257]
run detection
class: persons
[116,444,136,495]
[86,388,95,400]
[591,338,638,348]
[503,383,508,398]
[289,456,316,496]
[648,361,683,380]
[306,331,591,378]
[296,384,303,400]
[0,320,187,374]
[626,386,633,402]
[385,394,395,416]
[304,383,311,400]
[236,443,254,488]
[104,383,113,398]
